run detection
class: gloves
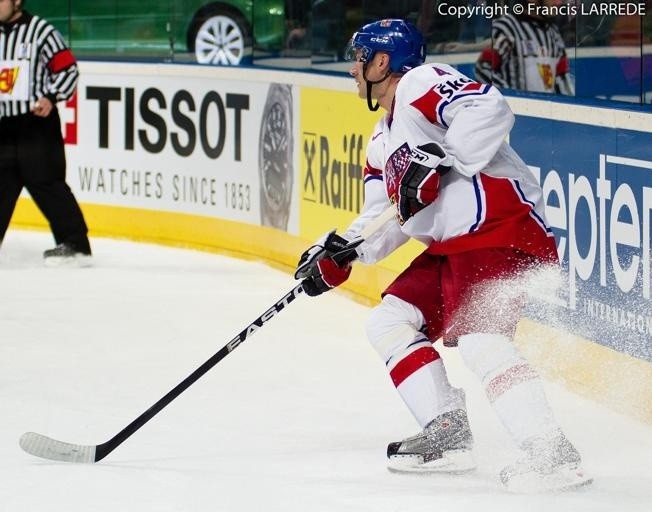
[292,226,358,298]
[385,137,454,227]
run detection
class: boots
[39,238,95,261]
[501,415,583,485]
[386,389,478,465]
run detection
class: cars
[16,0,288,69]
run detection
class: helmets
[343,17,429,74]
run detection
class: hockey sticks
[21,205,399,464]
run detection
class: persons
[472,0,576,99]
[0,0,94,260]
[292,18,599,496]
